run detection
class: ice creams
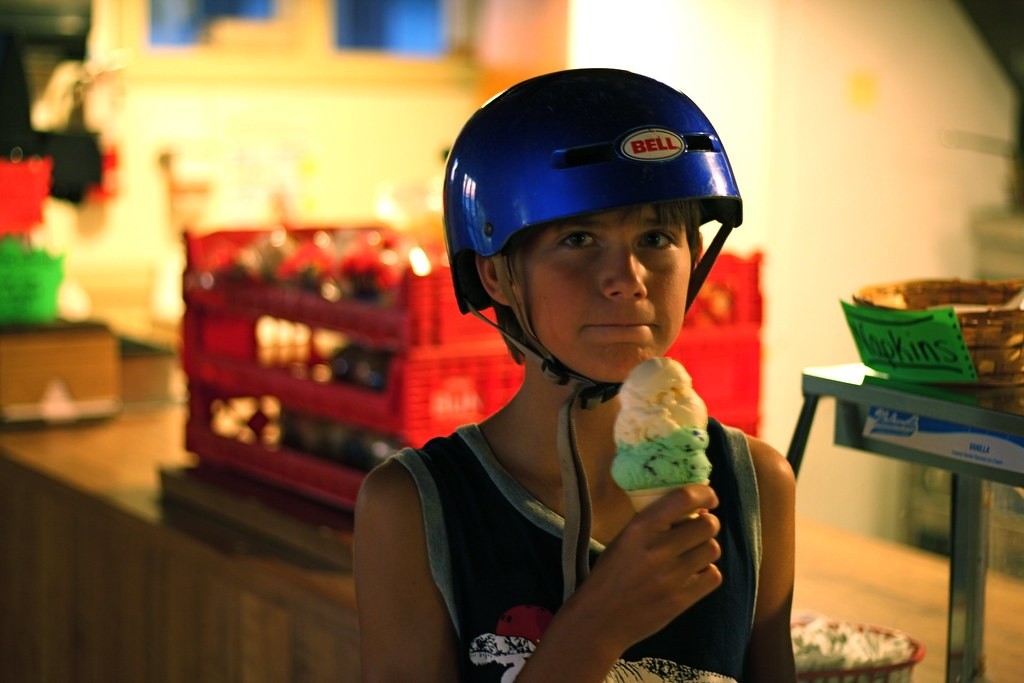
[609,356,713,525]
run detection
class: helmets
[443,66,742,315]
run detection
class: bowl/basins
[790,619,921,682]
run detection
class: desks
[784,363,1023,682]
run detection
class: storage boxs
[181,223,765,522]
[0,154,130,422]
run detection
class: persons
[353,67,795,682]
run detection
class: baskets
[852,275,1024,385]
